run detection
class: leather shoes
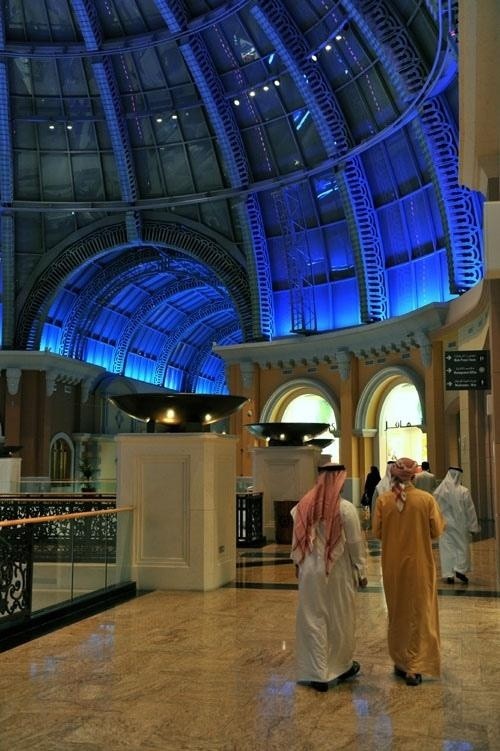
[447,572,468,584]
[395,665,422,686]
[311,660,360,691]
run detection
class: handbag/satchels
[361,492,368,506]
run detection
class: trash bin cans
[273,501,299,545]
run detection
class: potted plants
[75,437,103,492]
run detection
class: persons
[372,461,398,544]
[432,467,483,585]
[361,466,381,523]
[414,461,437,496]
[371,457,445,687]
[288,463,368,692]
